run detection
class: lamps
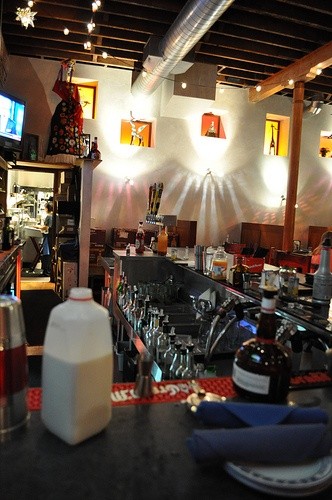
[15,0,38,29]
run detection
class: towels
[195,399,331,433]
[184,422,331,470]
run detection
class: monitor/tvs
[0,91,27,152]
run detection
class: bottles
[82,136,101,159]
[102,219,332,403]
[40,287,115,446]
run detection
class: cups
[0,296,30,444]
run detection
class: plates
[222,453,332,498]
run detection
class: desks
[89,226,319,273]
[0,240,27,300]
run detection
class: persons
[40,204,59,274]
